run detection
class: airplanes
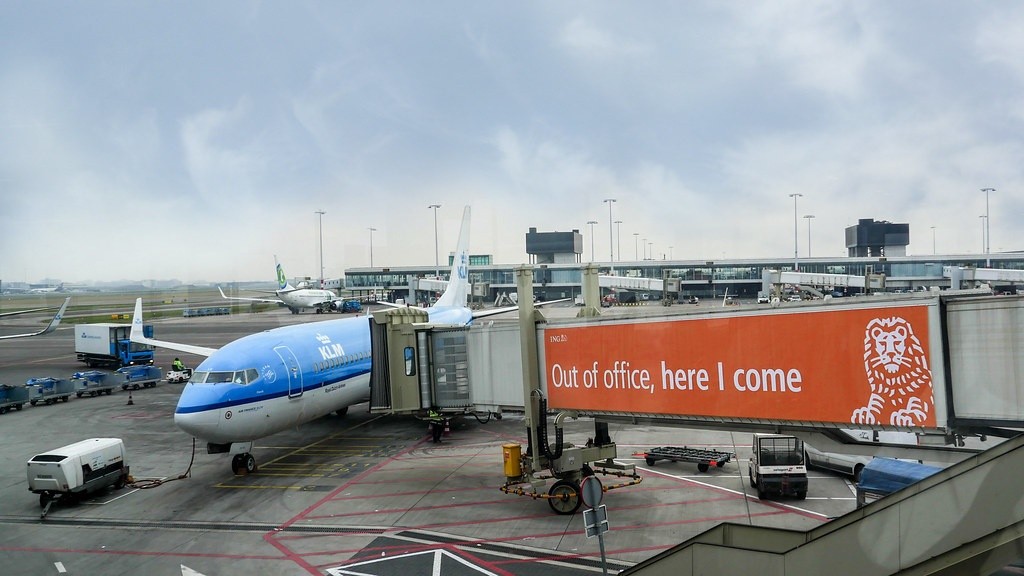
[217,253,377,315]
[126,202,574,481]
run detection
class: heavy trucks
[74,322,155,370]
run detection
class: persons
[428,407,444,443]
[174,358,184,370]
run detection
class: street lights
[428,204,442,277]
[980,187,996,268]
[603,198,617,262]
[587,221,598,262]
[366,226,377,268]
[648,242,653,261]
[633,233,639,261]
[614,220,622,262]
[789,193,803,271]
[669,246,674,261]
[642,238,648,260]
[803,214,816,258]
[930,227,936,254]
[315,211,326,283]
